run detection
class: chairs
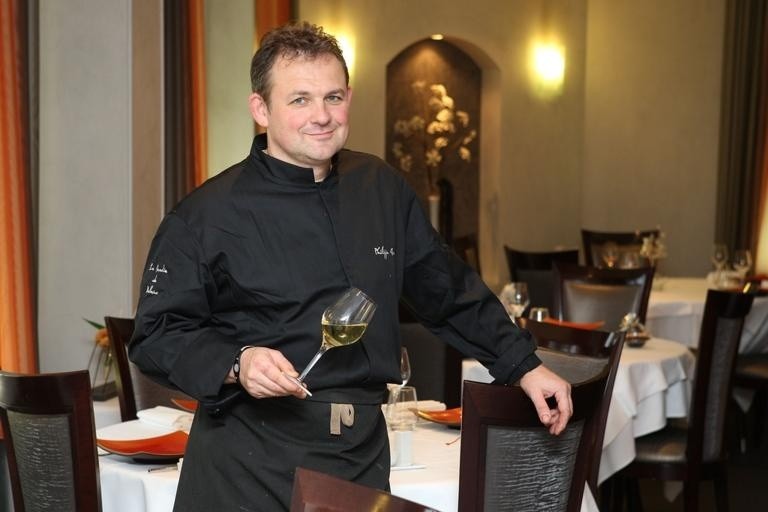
[580,228,661,266]
[514,317,627,496]
[458,360,609,512]
[553,258,654,333]
[621,282,757,512]
[101,316,195,423]
[288,466,444,512]
[500,242,578,318]
[0,367,102,512]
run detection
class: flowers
[76,316,113,390]
[393,73,478,190]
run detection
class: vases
[428,194,452,242]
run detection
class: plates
[573,266,650,284]
[541,316,607,337]
[739,271,768,284]
[94,428,188,462]
[412,405,462,427]
[173,396,204,413]
[733,287,766,295]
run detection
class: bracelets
[233,344,254,384]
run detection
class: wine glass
[708,244,729,279]
[282,284,375,397]
[731,249,753,284]
[384,385,421,431]
[383,346,410,389]
[498,280,549,322]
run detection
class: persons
[129,21,573,511]
